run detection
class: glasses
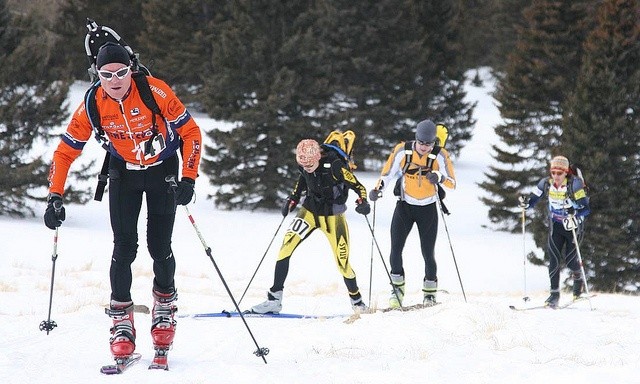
[98,66,131,80]
[552,171,563,175]
[418,140,434,146]
[300,164,314,168]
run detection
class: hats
[551,156,568,173]
[296,139,321,165]
[96,42,130,70]
[417,120,437,141]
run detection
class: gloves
[518,196,530,209]
[168,181,193,205]
[44,198,65,229]
[563,215,582,230]
[356,198,370,214]
[369,189,382,201]
[282,195,298,216]
[427,172,445,183]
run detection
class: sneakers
[151,278,178,344]
[351,297,365,310]
[545,290,559,305]
[252,289,283,314]
[573,279,582,295]
[105,294,136,355]
[422,278,437,303]
[389,269,406,307]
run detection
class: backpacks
[543,161,590,218]
[399,120,451,200]
[305,128,357,232]
[84,17,200,202]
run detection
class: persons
[518,153,590,306]
[249,139,371,315]
[43,40,202,360]
[369,119,456,308]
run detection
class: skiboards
[101,352,169,375]
[509,294,597,312]
[177,306,376,324]
[381,301,443,312]
